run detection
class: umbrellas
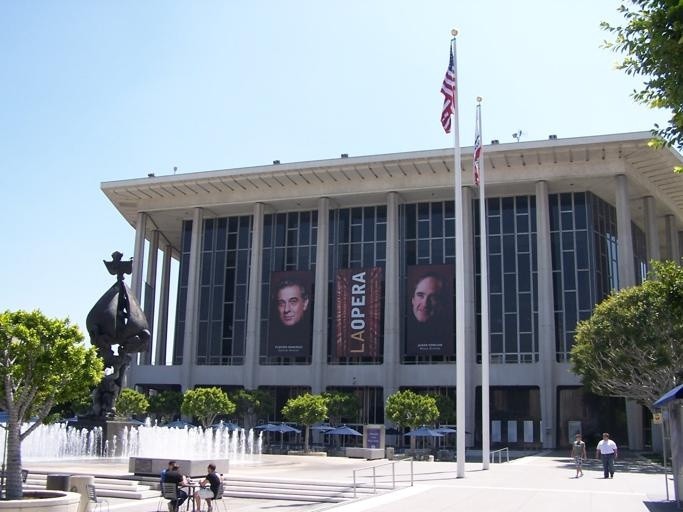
[433,426,456,448]
[403,426,444,451]
[127,417,362,449]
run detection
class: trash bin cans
[387,448,394,460]
[71,476,94,494]
[47,475,70,491]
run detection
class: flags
[438,38,458,136]
[472,106,483,189]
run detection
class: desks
[177,481,212,512]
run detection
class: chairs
[200,481,227,512]
[157,483,186,512]
[84,485,109,512]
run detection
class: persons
[194,464,220,512]
[164,463,187,511]
[160,460,176,491]
[404,272,452,355]
[595,432,617,478]
[570,433,586,478]
[269,279,309,356]
